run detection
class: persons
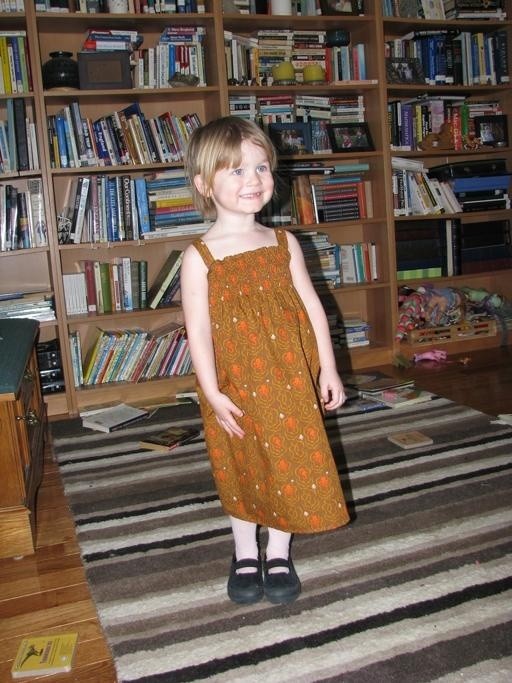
[182,117,348,604]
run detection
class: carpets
[46,375,512,683]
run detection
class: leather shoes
[264,553,302,603]
[228,550,263,603]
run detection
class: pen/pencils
[395,394,443,408]
[147,406,160,419]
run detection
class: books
[11,632,79,679]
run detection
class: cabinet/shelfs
[0,0,510,423]
[0,318,47,560]
